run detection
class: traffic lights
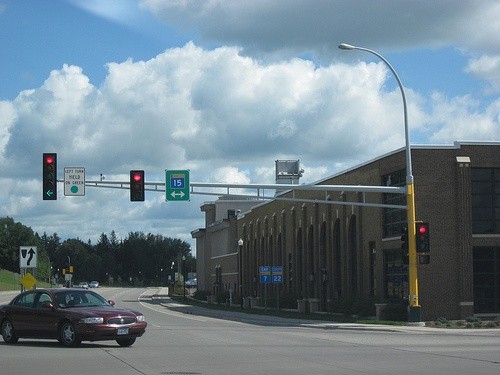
[400,226,409,249]
[415,221,430,253]
[403,255,409,265]
[130,169,146,202]
[417,255,430,265]
[43,153,57,200]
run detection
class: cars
[0,287,148,348]
[90,281,98,288]
[79,282,89,289]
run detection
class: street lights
[237,238,243,310]
[337,43,422,322]
[182,255,186,300]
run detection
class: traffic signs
[164,168,190,201]
